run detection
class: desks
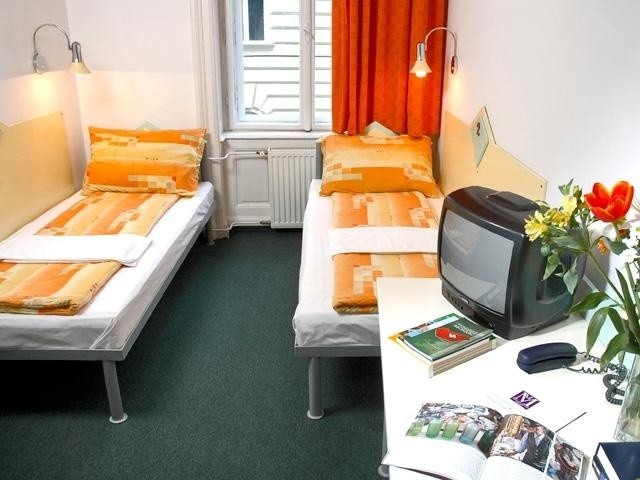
[376,275,640,480]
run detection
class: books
[478,381,587,433]
[592,457,605,480]
[383,399,585,480]
[596,440,640,480]
[387,313,496,380]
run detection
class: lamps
[31,22,93,76]
[409,26,460,78]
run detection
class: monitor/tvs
[437,186,588,339]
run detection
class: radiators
[267,147,317,229]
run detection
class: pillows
[80,126,207,198]
[319,134,440,197]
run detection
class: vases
[613,353,640,443]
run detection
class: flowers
[523,178,640,371]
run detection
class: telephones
[517,343,577,374]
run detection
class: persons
[507,421,550,472]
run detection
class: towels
[328,227,439,258]
[0,233,153,268]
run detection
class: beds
[0,181,217,425]
[291,178,446,421]
